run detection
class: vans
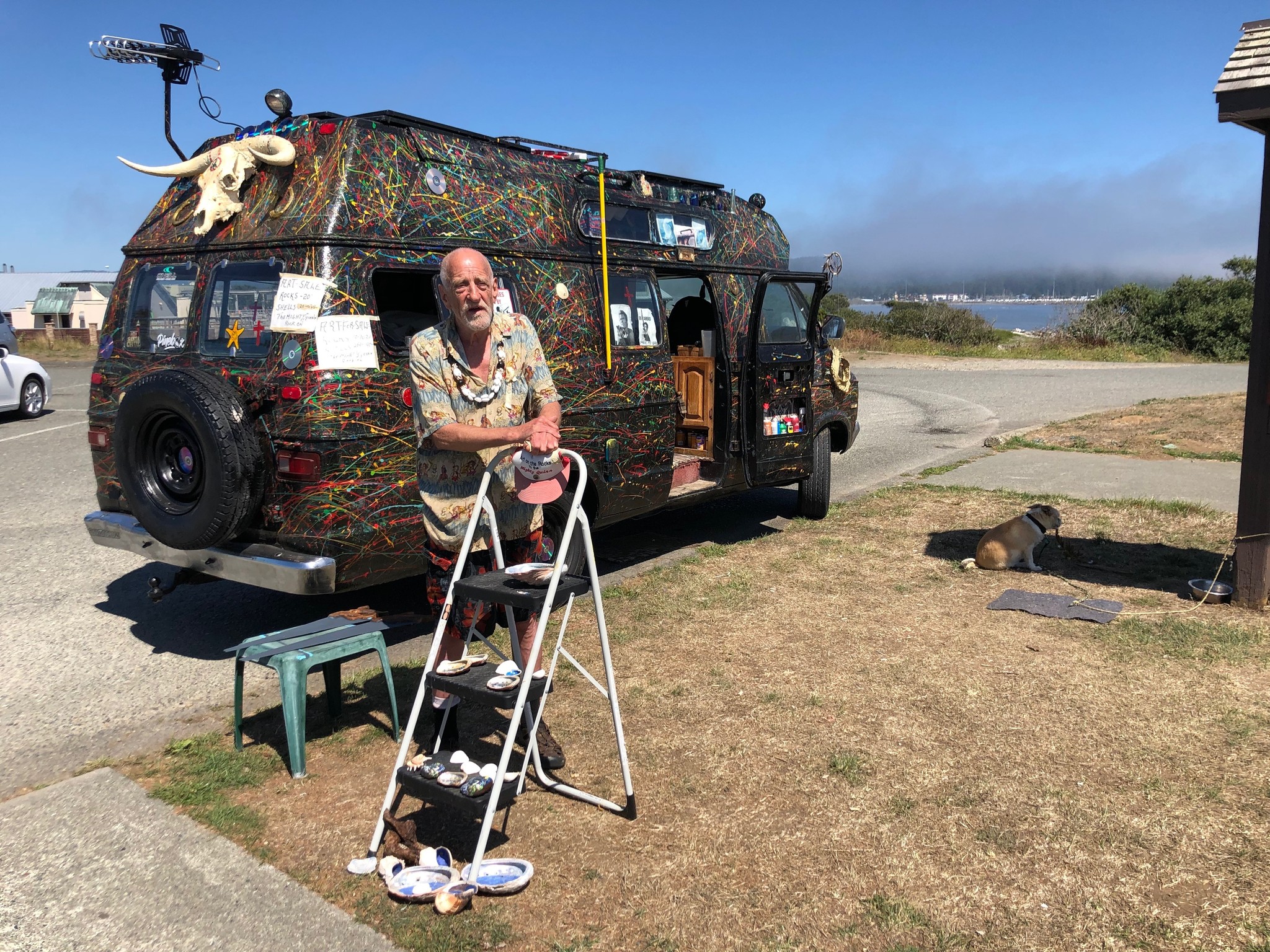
[82,116,861,616]
[0,311,18,356]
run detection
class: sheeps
[117,135,295,238]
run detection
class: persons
[405,247,569,770]
[642,323,650,342]
[615,310,635,346]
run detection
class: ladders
[363,444,637,890]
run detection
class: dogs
[961,504,1062,571]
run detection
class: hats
[512,444,571,505]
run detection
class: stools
[233,608,402,780]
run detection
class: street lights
[105,266,109,284]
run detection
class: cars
[0,348,53,419]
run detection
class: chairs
[669,291,724,357]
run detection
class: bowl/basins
[1189,579,1234,605]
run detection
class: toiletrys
[763,407,806,436]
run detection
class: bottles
[780,421,787,434]
[770,415,780,435]
[785,422,793,434]
[799,408,806,432]
[790,414,799,433]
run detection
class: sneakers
[429,713,461,752]
[519,718,567,769]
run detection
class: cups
[764,420,772,436]
